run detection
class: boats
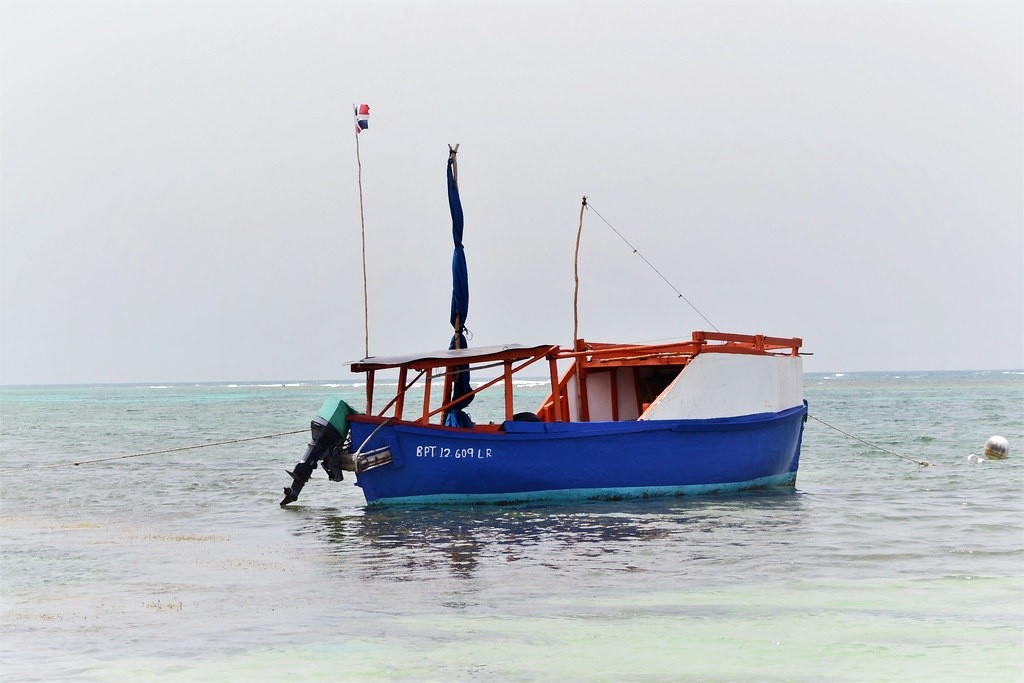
[278,142,811,514]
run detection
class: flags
[353,104,370,134]
